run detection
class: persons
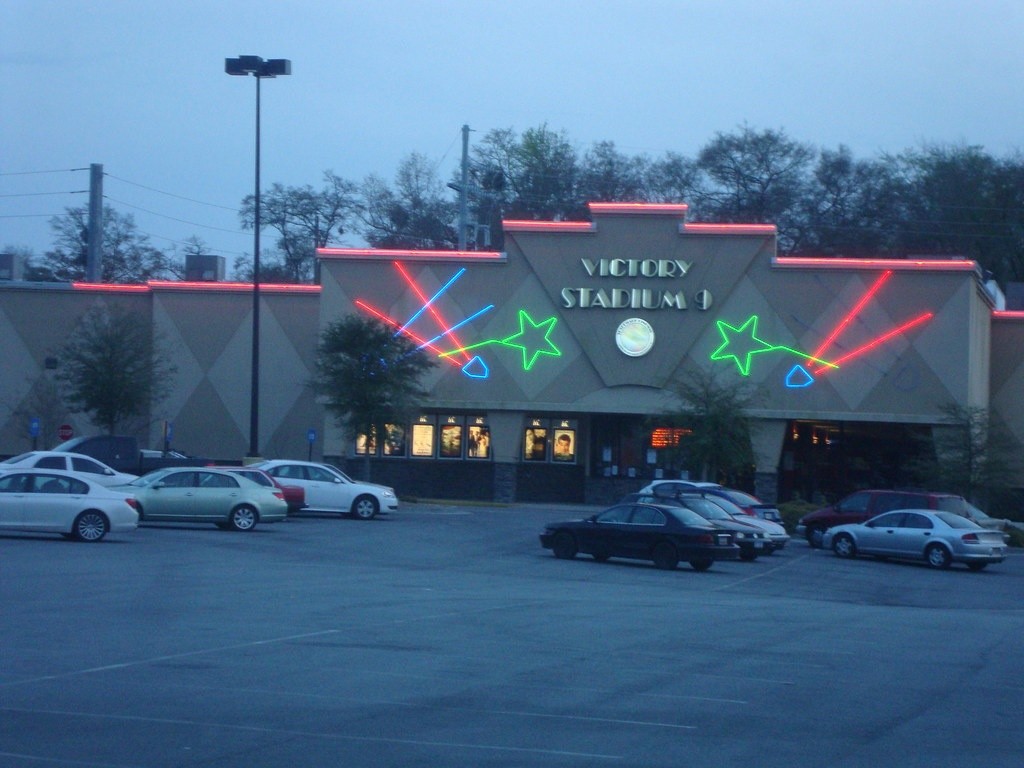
[469,430,489,457]
[555,435,574,457]
[525,429,546,460]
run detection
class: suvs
[799,489,970,547]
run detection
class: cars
[248,459,398,521]
[0,469,141,542]
[823,510,1011,570]
[0,450,309,532]
[966,503,1010,544]
[539,503,720,571]
[621,480,790,560]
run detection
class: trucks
[52,436,242,477]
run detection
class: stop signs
[58,425,73,440]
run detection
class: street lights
[224,55,291,466]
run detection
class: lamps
[811,436,818,443]
[45,357,57,369]
